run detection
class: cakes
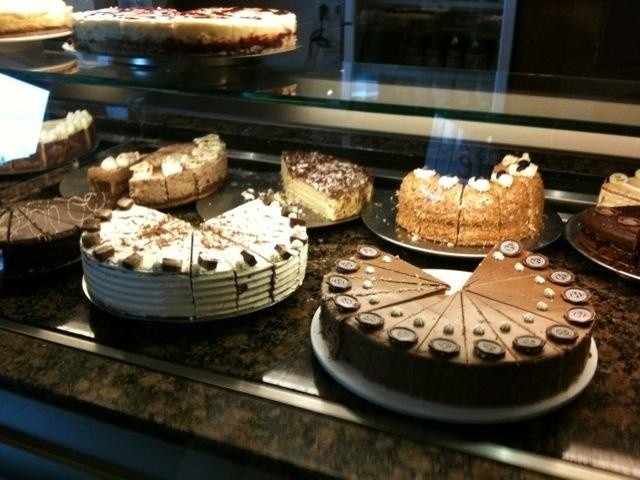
[87,132,229,207]
[0,197,94,275]
[574,168,639,276]
[79,189,310,324]
[280,149,376,222]
[319,237,598,409]
[394,152,547,250]
[1,2,300,56]
[1,109,97,173]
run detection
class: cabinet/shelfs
[0,47,640,480]
